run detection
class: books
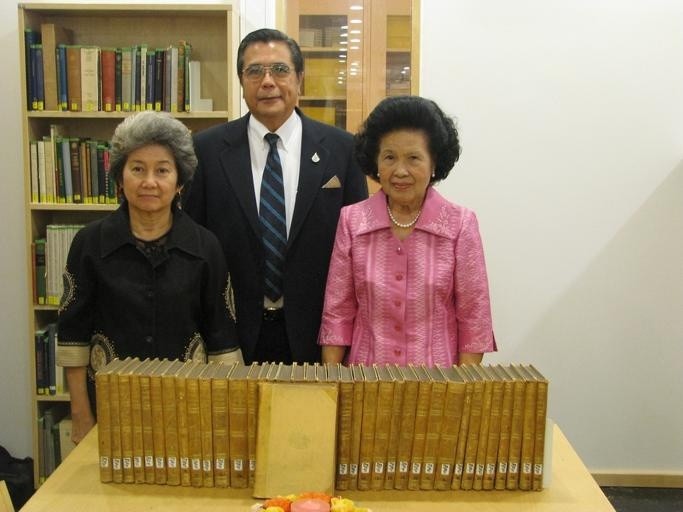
[28,123,123,204]
[31,222,88,306]
[95,358,548,503]
[23,27,201,114]
[35,407,76,485]
[34,322,68,395]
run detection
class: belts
[264,307,285,320]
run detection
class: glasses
[243,63,295,81]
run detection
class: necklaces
[385,202,421,228]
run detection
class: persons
[315,93,502,368]
[177,27,376,364]
[52,109,247,446]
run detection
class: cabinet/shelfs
[17,2,239,485]
[269,3,419,199]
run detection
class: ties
[258,133,289,304]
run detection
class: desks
[16,420,618,511]
